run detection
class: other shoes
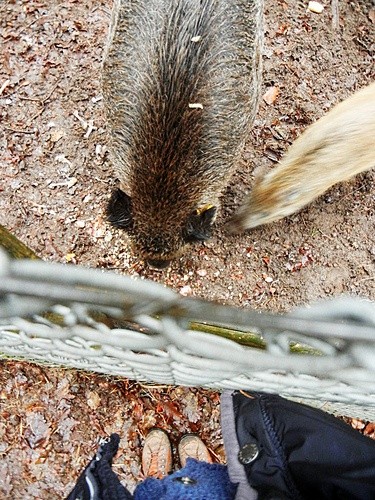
[176,432,214,467]
[141,428,174,479]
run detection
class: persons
[132,426,236,499]
